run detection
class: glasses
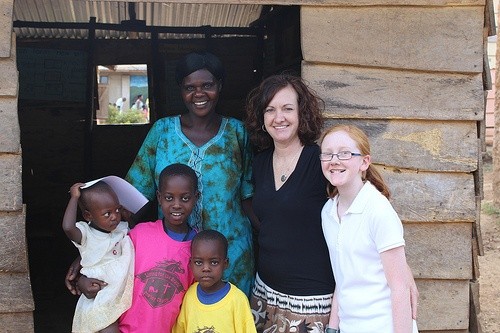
[319,151,362,161]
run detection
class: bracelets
[324,327,340,333]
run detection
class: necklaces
[274,144,302,182]
[162,216,191,241]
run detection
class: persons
[246,73,420,333]
[62,182,136,333]
[77,162,207,333]
[170,228,258,333]
[315,124,420,333]
[107,92,150,122]
[65,51,259,304]
